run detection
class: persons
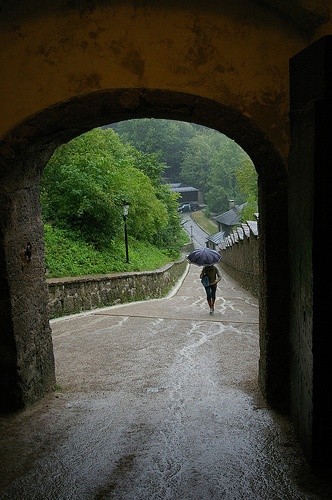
[200,263,222,314]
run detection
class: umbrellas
[187,248,222,267]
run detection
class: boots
[210,299,215,313]
[207,299,212,309]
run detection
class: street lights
[122,200,131,263]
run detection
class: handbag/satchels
[202,276,210,287]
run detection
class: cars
[177,203,200,212]
